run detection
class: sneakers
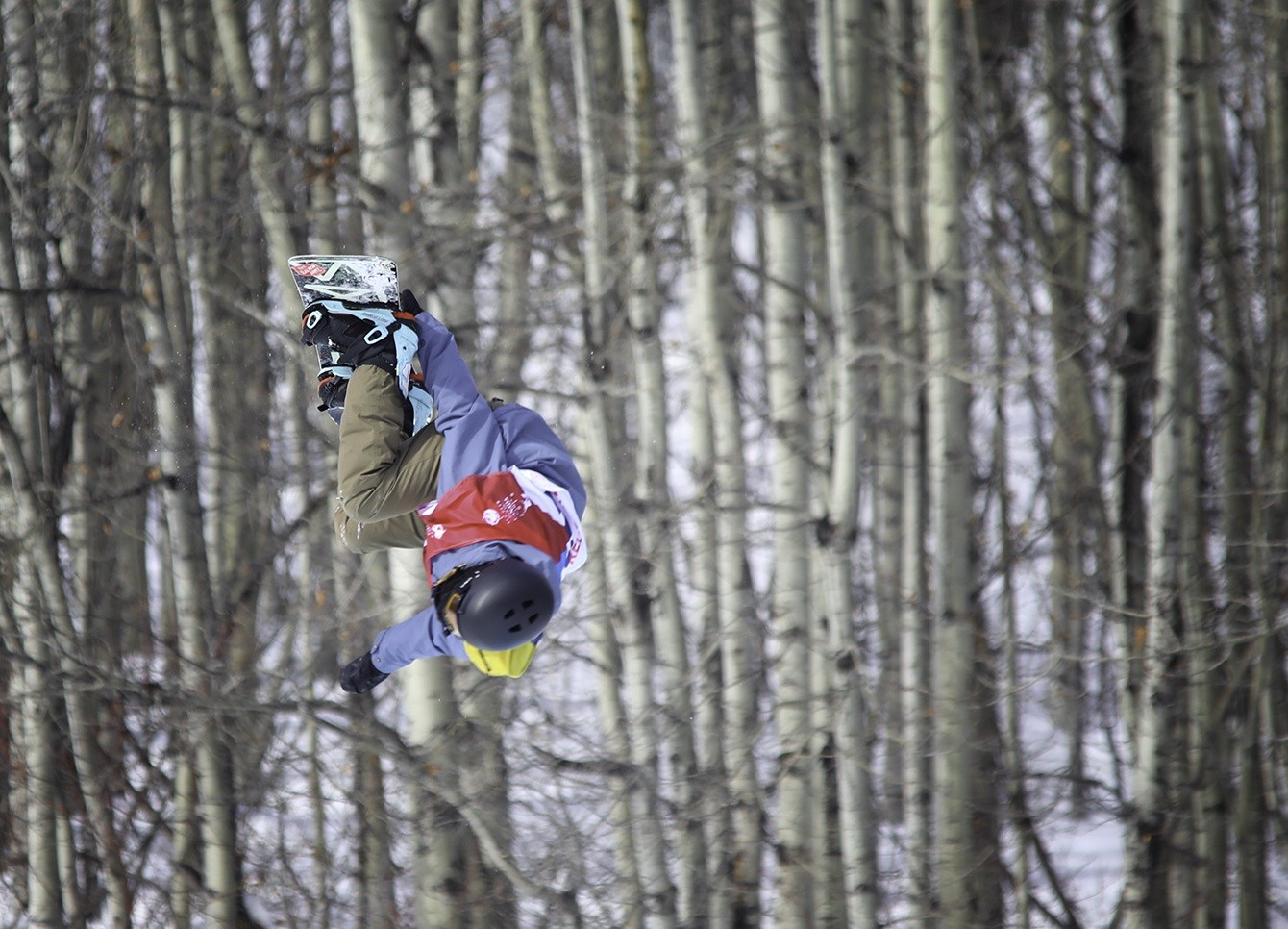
[302,308,418,360]
[317,376,428,434]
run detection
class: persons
[297,290,589,694]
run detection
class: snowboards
[288,253,405,433]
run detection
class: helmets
[455,558,555,652]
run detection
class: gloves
[399,289,424,316]
[339,651,390,697]
